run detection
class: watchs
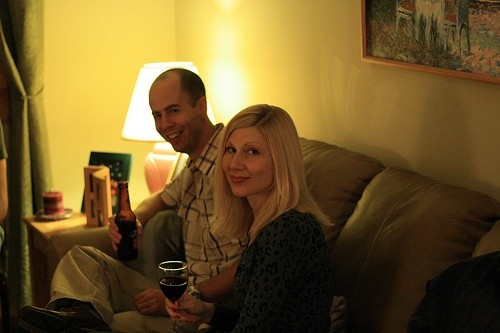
[187,285,203,301]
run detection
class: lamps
[120,61,216,190]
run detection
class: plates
[36,208,73,220]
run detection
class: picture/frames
[80,151,132,213]
[360,0,500,85]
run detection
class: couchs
[299,137,500,333]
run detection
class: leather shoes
[20,304,113,333]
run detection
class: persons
[406,249,500,333]
[0,118,8,254]
[165,105,336,333]
[15,69,251,333]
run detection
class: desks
[23,213,111,286]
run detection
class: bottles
[114,180,139,261]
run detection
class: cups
[42,191,64,217]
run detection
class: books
[82,163,113,227]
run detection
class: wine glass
[157,260,189,333]
